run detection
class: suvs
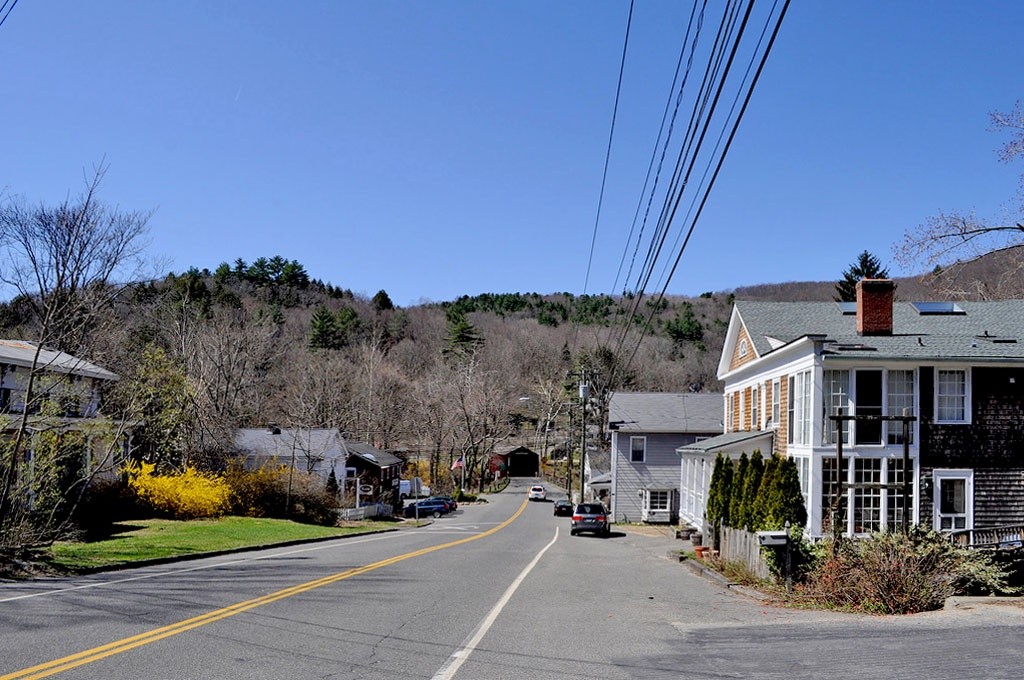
[528,484,547,502]
[571,502,613,538]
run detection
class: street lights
[518,397,573,500]
[546,428,570,489]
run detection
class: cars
[553,498,574,518]
[398,479,458,520]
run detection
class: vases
[695,546,710,559]
[690,534,701,545]
[702,549,719,562]
[680,528,693,540]
